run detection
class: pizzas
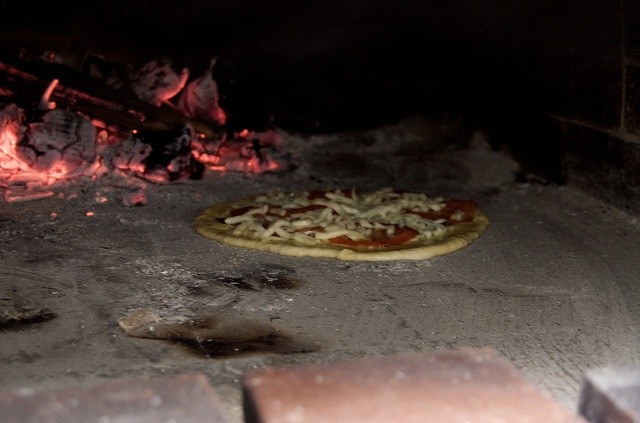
[191,185,490,262]
